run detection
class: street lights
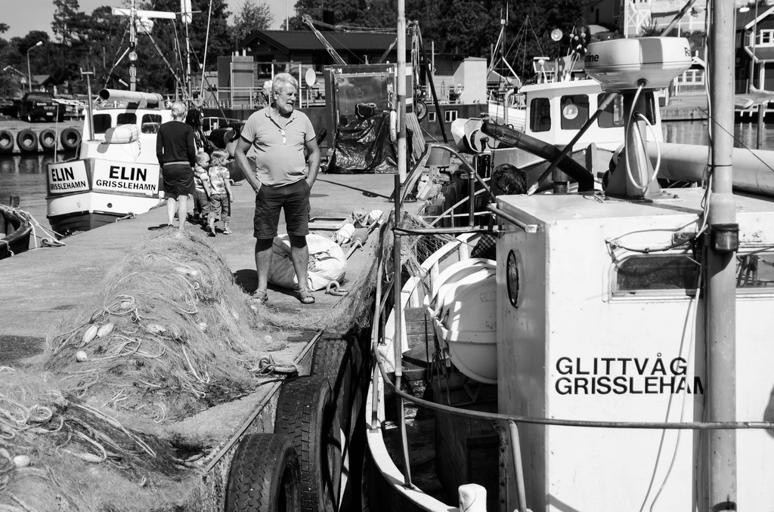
[26,41,42,92]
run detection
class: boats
[0,196,32,259]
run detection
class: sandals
[252,289,269,306]
[295,288,316,304]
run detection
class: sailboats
[362,0,773,512]
[46,0,258,239]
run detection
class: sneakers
[197,216,233,237]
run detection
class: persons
[234,72,321,307]
[205,148,233,237]
[155,101,195,231]
[192,151,212,230]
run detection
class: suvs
[13,90,66,121]
